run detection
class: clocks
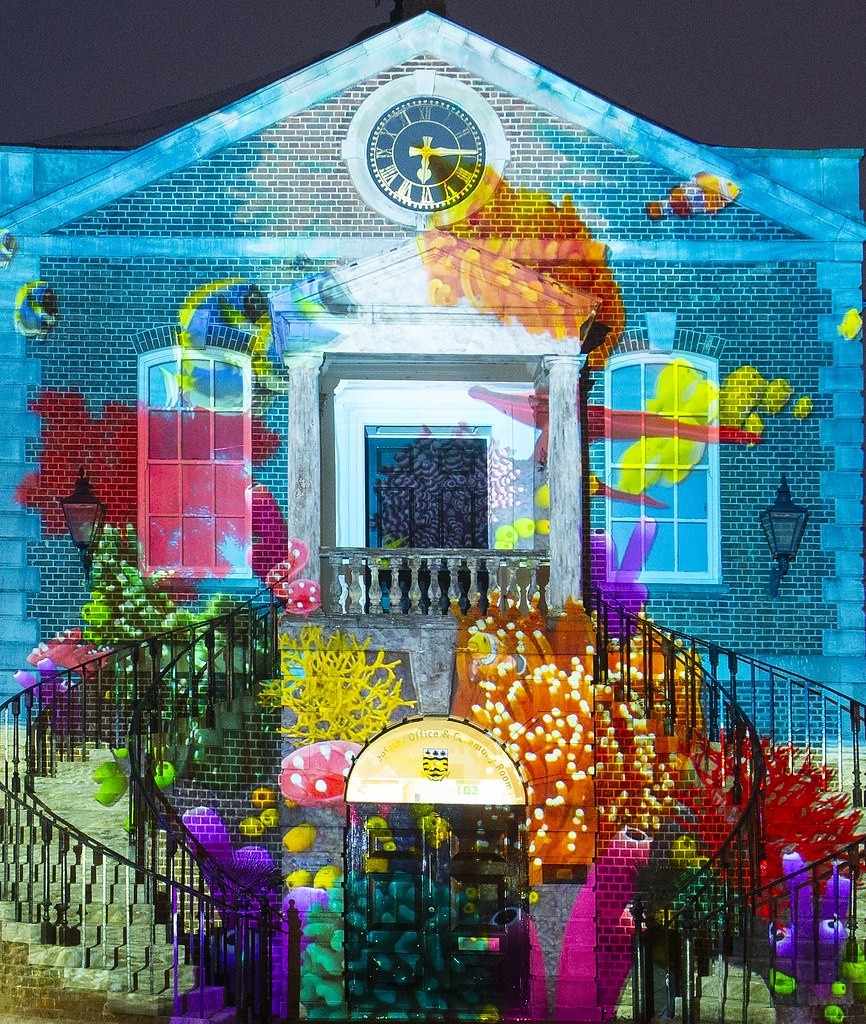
[340,69,511,234]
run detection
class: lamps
[760,472,810,597]
[61,466,108,583]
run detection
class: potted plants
[631,859,698,1016]
